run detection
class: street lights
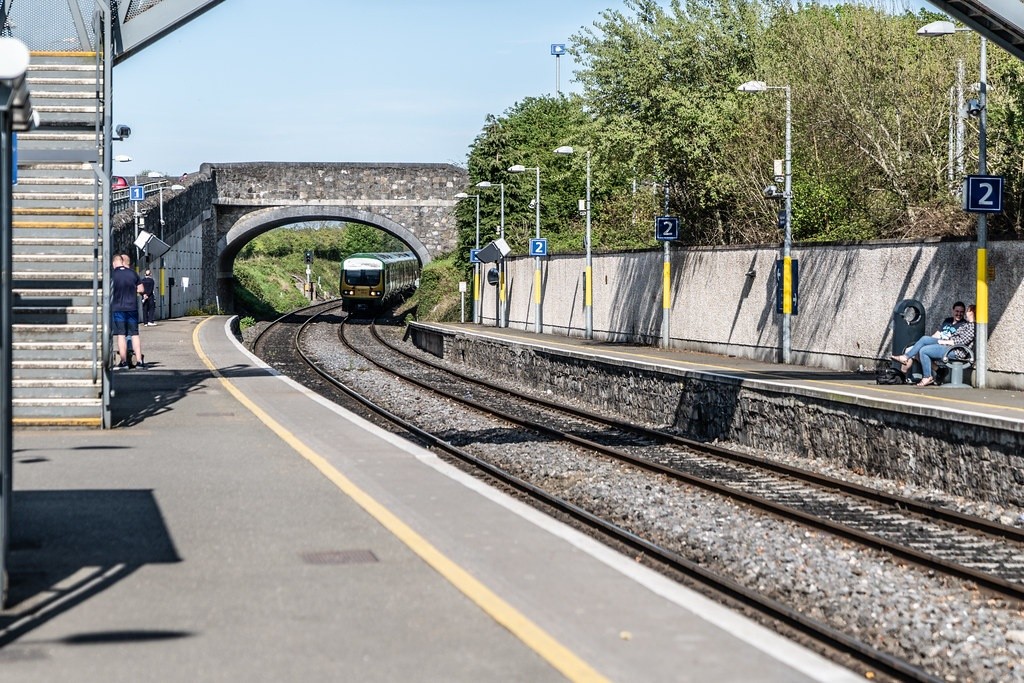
[553,146,593,339]
[476,181,505,327]
[135,172,164,323]
[736,80,792,365]
[455,193,479,324]
[916,20,987,389]
[160,185,186,319]
[508,165,541,333]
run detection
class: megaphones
[529,199,535,208]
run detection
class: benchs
[901,341,976,389]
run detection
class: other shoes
[143,324,147,327]
[136,361,145,369]
[148,322,158,326]
[110,359,129,370]
[934,366,948,384]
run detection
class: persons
[891,301,976,386]
[111,255,144,369]
[139,270,158,326]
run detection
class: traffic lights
[304,251,313,264]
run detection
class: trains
[340,251,419,318]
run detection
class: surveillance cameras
[141,208,146,214]
[140,218,144,228]
[763,186,776,196]
[161,221,165,225]
[968,99,980,116]
[116,125,131,138]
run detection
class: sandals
[890,354,908,366]
[916,378,935,386]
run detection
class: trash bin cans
[891,299,925,382]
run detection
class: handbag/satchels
[874,362,905,386]
